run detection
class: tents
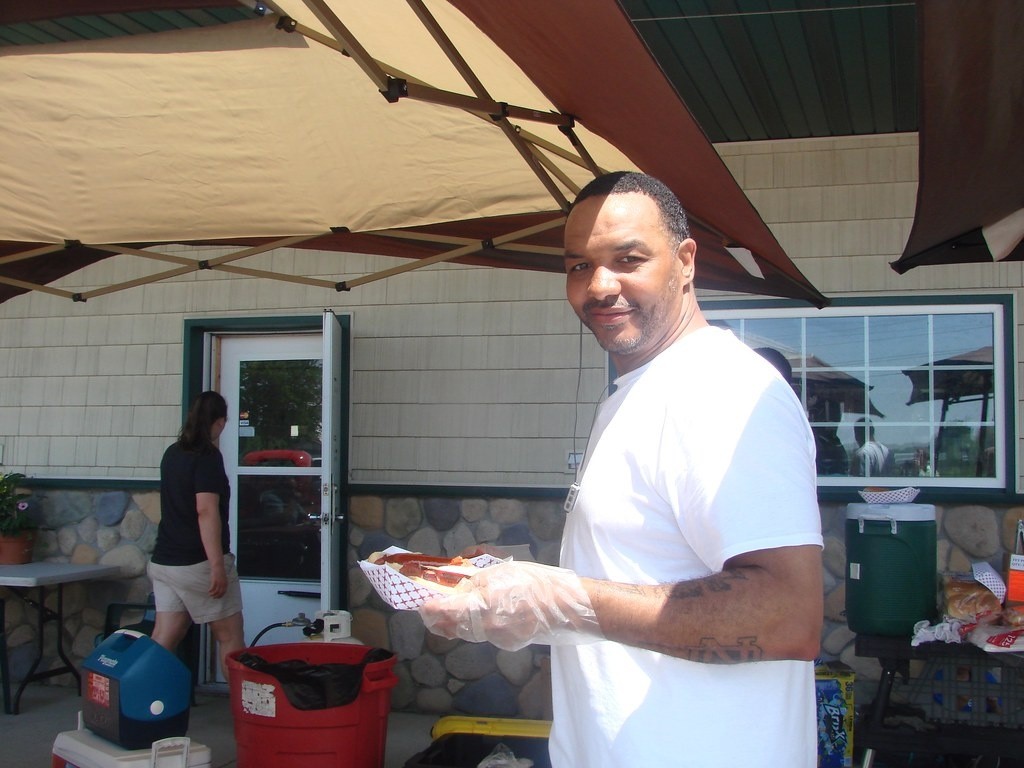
[715,325,886,418]
[0,0,826,310]
[902,346,994,477]
[887,1,1024,277]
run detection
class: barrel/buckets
[845,503,937,637]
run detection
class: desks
[0,562,120,715]
[853,633,1024,768]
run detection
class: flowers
[0,463,48,541]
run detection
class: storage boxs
[1002,552,1024,608]
[815,661,855,768]
[51,710,211,768]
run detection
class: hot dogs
[368,549,481,594]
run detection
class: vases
[0,529,35,565]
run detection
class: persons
[753,347,792,385]
[148,390,248,682]
[851,417,896,478]
[418,171,827,768]
[813,399,849,477]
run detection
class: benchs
[855,719,1024,760]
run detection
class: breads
[939,575,1024,626]
